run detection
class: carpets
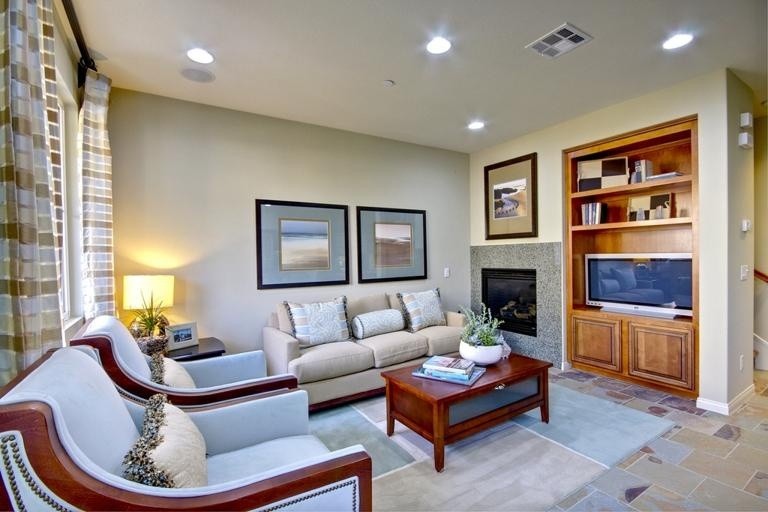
[308,347,680,512]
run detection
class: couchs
[261,291,471,415]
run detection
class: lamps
[122,272,176,311]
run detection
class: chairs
[69,313,297,407]
[0,345,372,512]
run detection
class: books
[581,202,602,225]
[631,159,682,184]
[411,355,487,387]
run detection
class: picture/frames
[165,322,198,352]
[483,153,539,241]
[356,205,428,284]
[254,199,351,290]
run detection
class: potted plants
[458,304,505,368]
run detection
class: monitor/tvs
[585,252,692,319]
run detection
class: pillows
[151,349,199,390]
[397,288,449,333]
[350,308,404,340]
[128,396,206,491]
[283,296,352,349]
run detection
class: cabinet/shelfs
[561,113,700,399]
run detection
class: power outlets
[444,268,449,277]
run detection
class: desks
[165,335,226,361]
